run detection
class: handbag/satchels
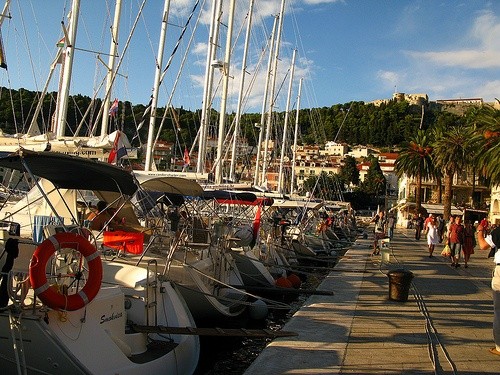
[492,265,500,291]
[440,245,452,258]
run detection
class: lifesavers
[29,233,103,311]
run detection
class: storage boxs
[102,231,144,255]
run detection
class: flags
[0,26,8,69]
[107,130,128,163]
[51,35,66,63]
[183,145,191,166]
[108,100,118,116]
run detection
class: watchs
[476,230,482,234]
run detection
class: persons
[78,200,358,242]
[371,209,398,255]
[476,223,500,356]
[406,209,500,268]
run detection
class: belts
[496,263,500,265]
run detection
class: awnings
[388,203,404,213]
[420,204,464,215]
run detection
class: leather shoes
[490,347,500,355]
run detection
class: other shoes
[464,264,468,268]
[429,254,434,258]
[451,262,458,267]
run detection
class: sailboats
[0,0,365,375]
